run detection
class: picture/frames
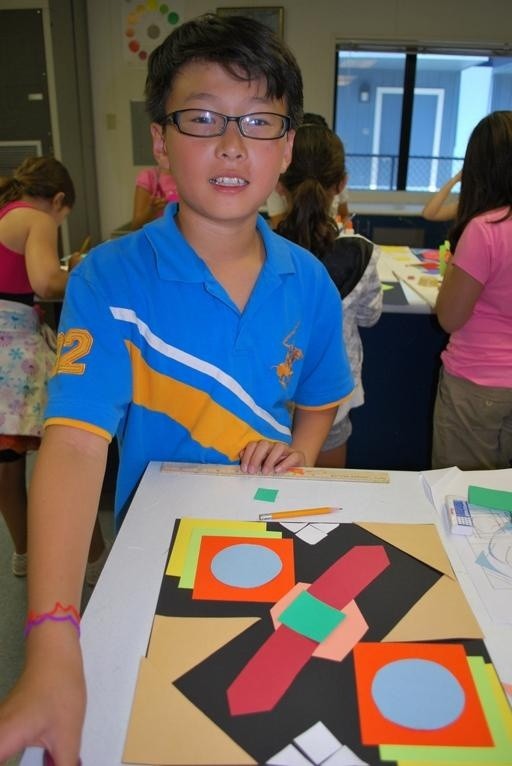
[217,5,285,39]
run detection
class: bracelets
[23,601,82,641]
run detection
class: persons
[421,168,463,222]
[129,165,179,230]
[270,124,383,468]
[267,112,349,232]
[0,154,110,586]
[1,12,357,766]
[427,109,512,470]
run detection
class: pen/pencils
[80,235,92,254]
[339,213,356,233]
[259,507,343,520]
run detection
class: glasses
[163,108,290,141]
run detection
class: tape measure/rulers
[159,460,389,482]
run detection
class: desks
[33,242,438,329]
[18,462,512,766]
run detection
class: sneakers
[85,541,115,586]
[13,551,30,576]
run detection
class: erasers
[446,496,474,534]
